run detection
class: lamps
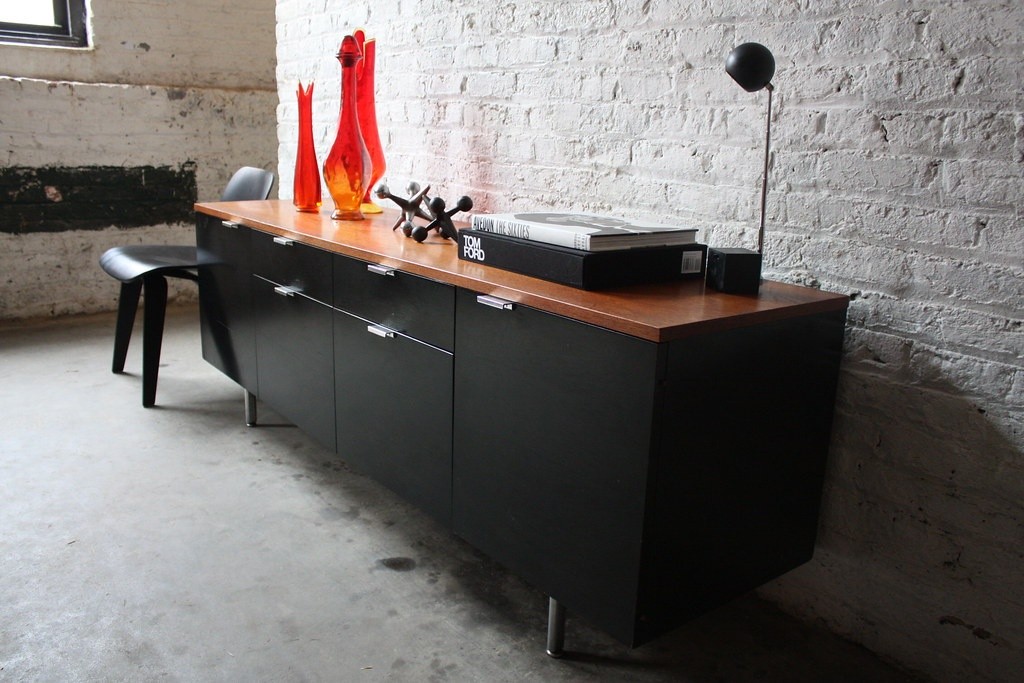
[707,41,776,298]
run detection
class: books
[458,208,711,293]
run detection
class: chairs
[99,165,275,408]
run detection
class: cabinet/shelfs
[262,197,336,455]
[455,222,852,657]
[193,198,264,426]
[334,203,455,533]
